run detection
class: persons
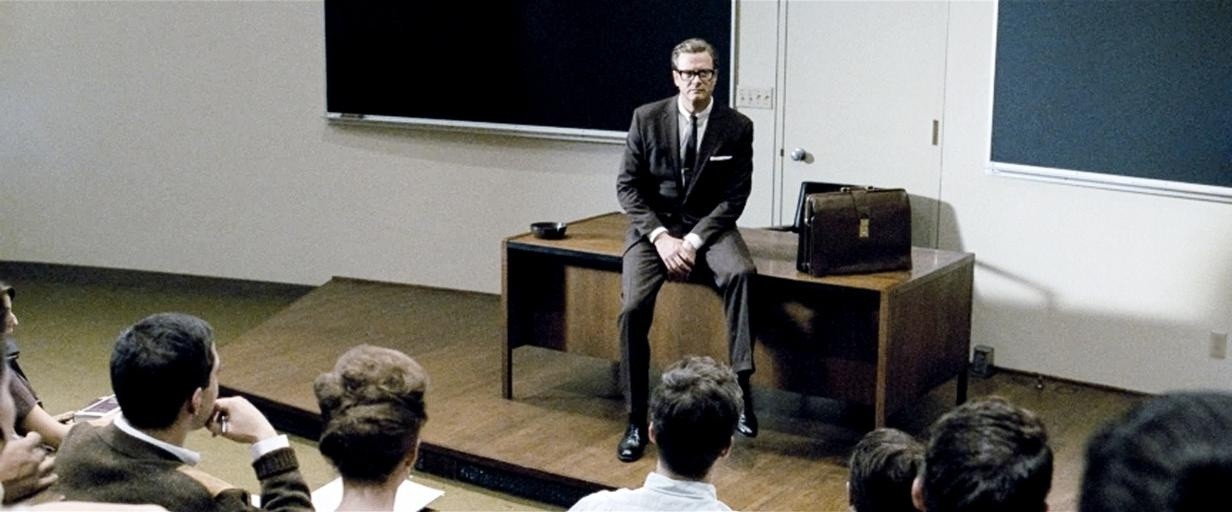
[1079,393,1230,509]
[566,356,743,511]
[847,428,924,511]
[55,313,309,512]
[0,430,61,512]
[0,286,120,452]
[910,398,1050,511]
[315,341,430,511]
[615,39,758,462]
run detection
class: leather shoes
[738,406,759,439]
[618,422,647,462]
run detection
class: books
[309,471,444,512]
[71,395,122,425]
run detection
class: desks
[501,211,975,429]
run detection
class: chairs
[761,180,881,233]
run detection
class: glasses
[675,68,717,79]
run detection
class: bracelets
[681,240,698,254]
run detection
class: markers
[340,112,364,120]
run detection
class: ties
[685,118,698,181]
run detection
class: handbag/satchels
[795,189,910,273]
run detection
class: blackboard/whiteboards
[323,0,741,149]
[986,0,1232,206]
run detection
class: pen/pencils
[221,415,226,433]
